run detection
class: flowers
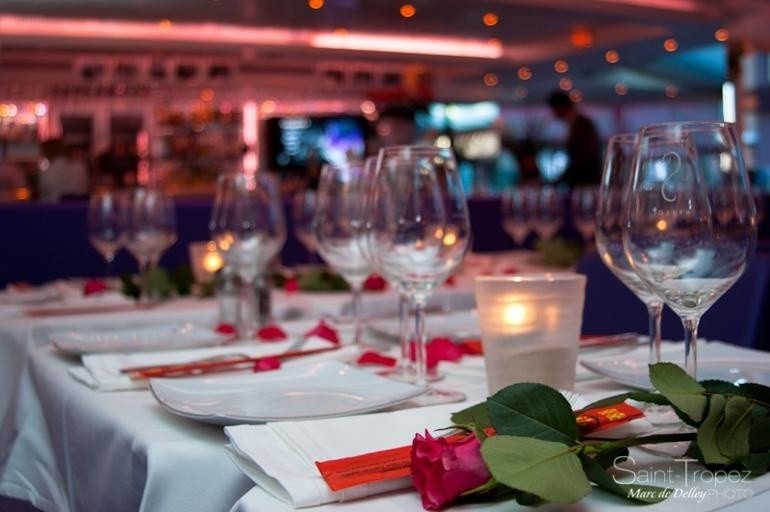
[410,361,770,511]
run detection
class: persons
[546,87,601,185]
[36,138,86,202]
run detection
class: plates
[366,304,483,345]
[148,355,429,427]
[580,337,770,396]
[55,321,235,357]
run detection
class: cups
[475,275,587,402]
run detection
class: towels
[67,329,395,393]
[222,389,589,506]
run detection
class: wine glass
[363,144,470,408]
[623,122,758,458]
[86,188,129,305]
[499,185,531,266]
[358,155,447,383]
[528,187,565,263]
[313,164,397,364]
[123,186,176,309]
[208,172,287,347]
[571,188,596,273]
[593,134,686,426]
